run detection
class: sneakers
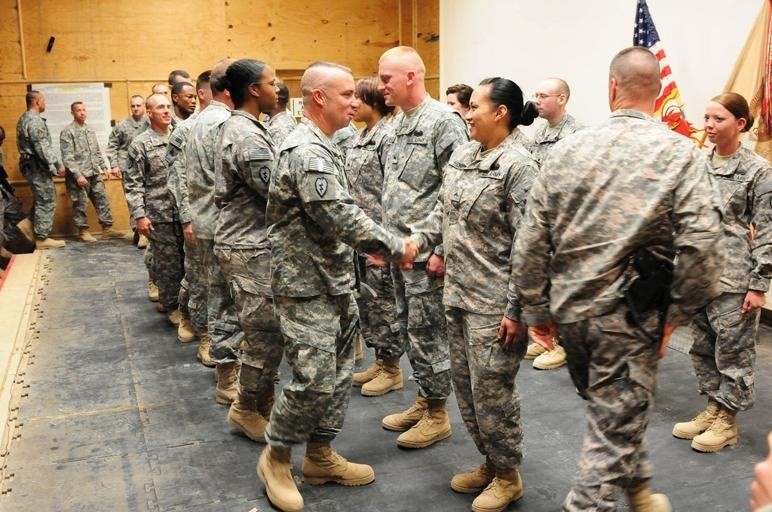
[523,337,559,360]
[450,455,496,495]
[35,225,149,252]
[396,405,452,448]
[471,464,525,512]
[532,343,569,370]
[146,276,217,368]
[381,396,429,432]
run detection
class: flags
[723,1,772,164]
[634,0,700,142]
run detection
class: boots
[628,484,673,512]
[254,443,305,512]
[671,396,723,440]
[211,360,241,405]
[352,358,384,388]
[227,393,271,443]
[300,441,376,487]
[255,382,277,422]
[691,405,739,453]
[360,356,404,398]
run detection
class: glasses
[535,94,562,99]
[249,80,281,87]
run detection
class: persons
[670,92,771,453]
[16,90,66,249]
[105,45,583,512]
[513,47,724,511]
[59,102,129,243]
